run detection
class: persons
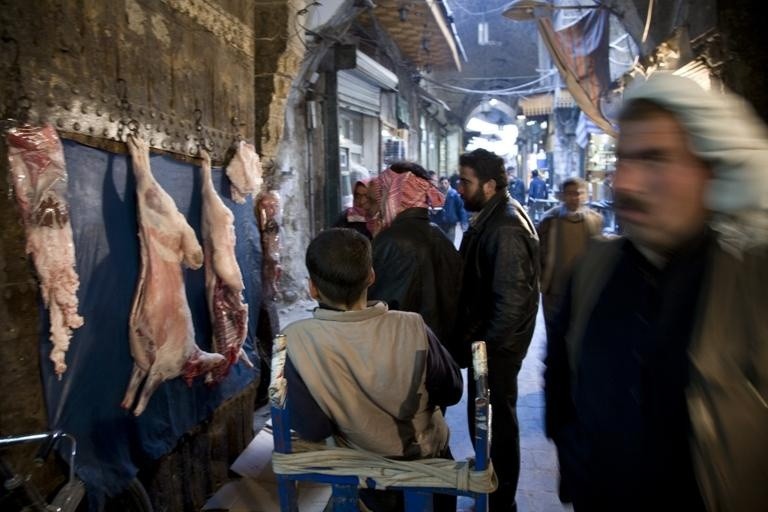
[428,168,469,244]
[366,163,472,369]
[336,179,379,240]
[596,171,613,228]
[448,147,543,512]
[506,165,543,220]
[281,227,465,512]
[546,70,767,510]
[536,176,604,303]
